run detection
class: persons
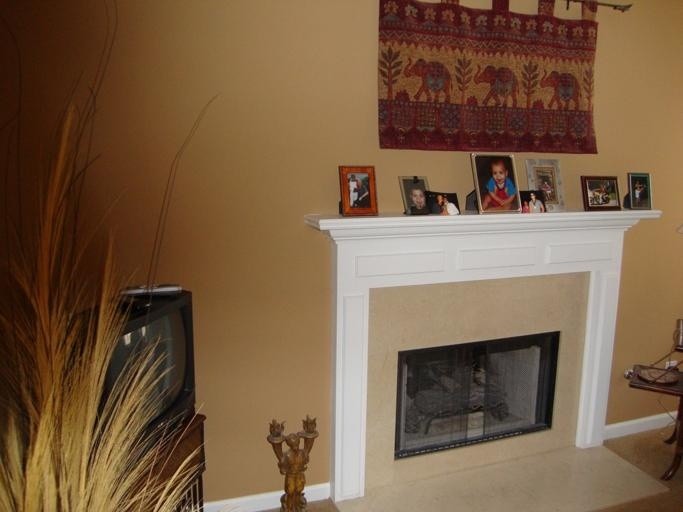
[521,201,529,213]
[632,179,648,208]
[588,190,600,204]
[408,183,430,214]
[480,161,516,210]
[434,195,445,216]
[442,196,460,215]
[598,184,609,204]
[346,173,369,208]
[528,192,544,213]
[538,176,556,202]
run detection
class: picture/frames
[623,173,654,210]
[338,165,378,217]
[398,175,431,215]
[465,151,522,213]
[524,158,566,213]
[580,175,622,211]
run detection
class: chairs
[623,319,682,480]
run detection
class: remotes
[118,282,185,296]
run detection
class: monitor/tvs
[60,290,197,482]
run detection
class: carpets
[331,447,669,512]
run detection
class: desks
[127,414,207,512]
